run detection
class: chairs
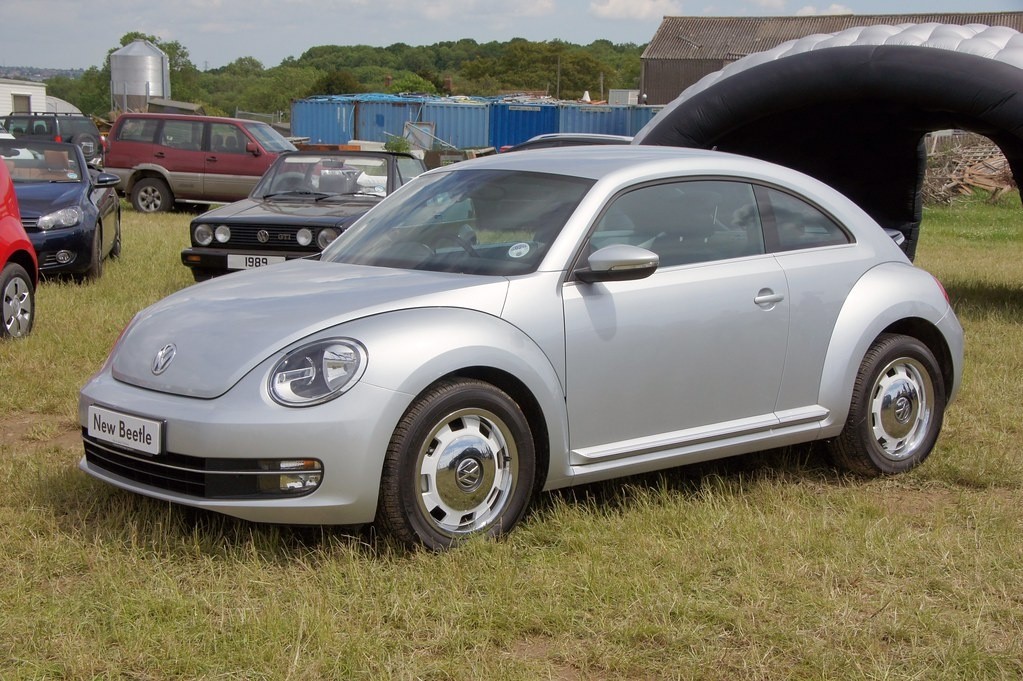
[211,135,229,152]
[225,136,241,153]
[319,175,347,193]
[649,212,717,269]
[37,149,77,181]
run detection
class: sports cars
[72,142,967,557]
[0,138,124,283]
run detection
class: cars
[448,132,635,232]
[0,156,39,341]
[180,151,432,282]
[0,120,75,163]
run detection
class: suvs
[103,111,360,213]
[1,111,106,163]
